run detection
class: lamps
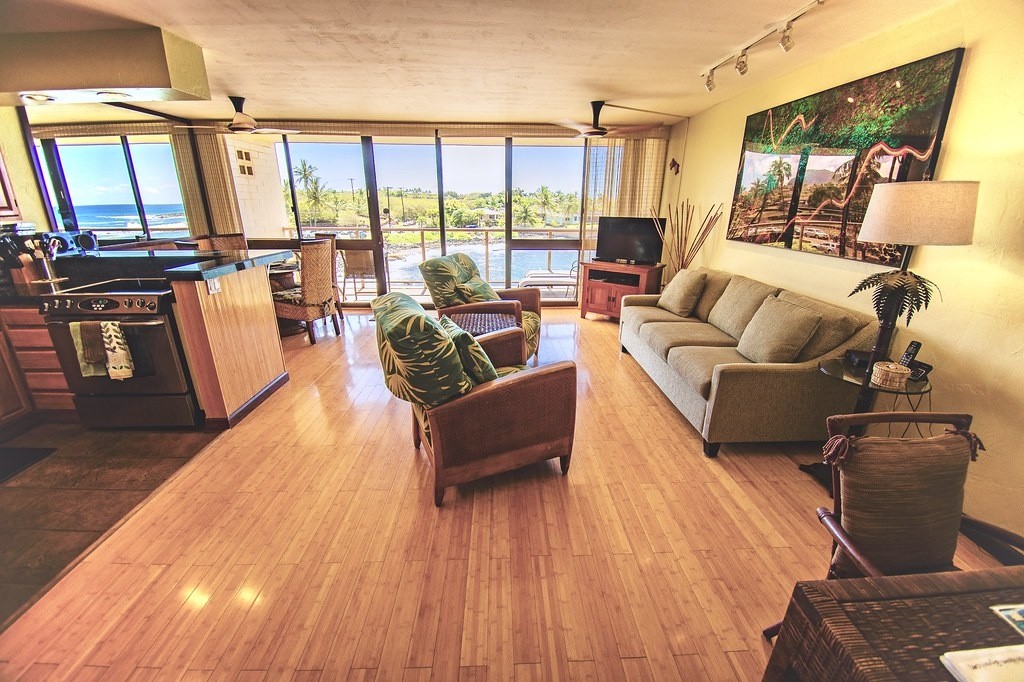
[777,21,795,53]
[799,180,980,499]
[704,69,716,92]
[734,50,748,76]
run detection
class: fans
[559,101,662,141]
[173,96,301,134]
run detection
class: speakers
[43,230,98,256]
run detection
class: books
[939,643,1024,682]
[989,603,1024,636]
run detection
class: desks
[452,311,518,336]
[762,564,1024,682]
[247,250,293,276]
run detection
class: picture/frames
[725,48,966,269]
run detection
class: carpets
[0,446,57,484]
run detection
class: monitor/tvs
[597,217,666,266]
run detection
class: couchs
[619,266,899,458]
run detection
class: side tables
[820,358,932,395]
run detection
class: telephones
[899,340,933,383]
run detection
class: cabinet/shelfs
[0,309,75,427]
[580,261,666,319]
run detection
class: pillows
[656,268,708,317]
[439,314,499,386]
[455,276,502,302]
[737,294,822,363]
[821,427,984,579]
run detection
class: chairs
[273,239,340,344]
[340,250,391,303]
[207,233,248,251]
[419,253,541,366]
[518,260,578,300]
[174,241,200,251]
[315,233,344,325]
[762,412,1024,638]
[370,292,577,507]
